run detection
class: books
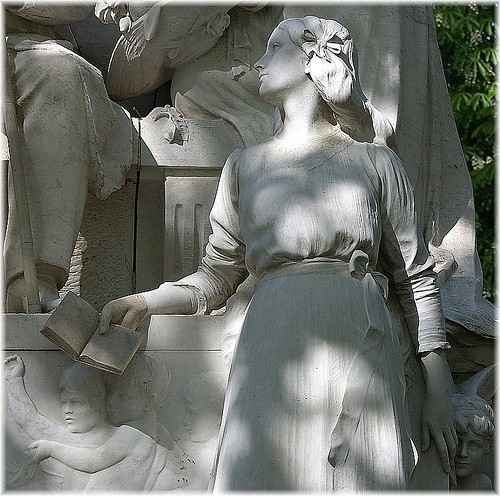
[38,290,143,377]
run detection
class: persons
[4,355,167,492]
[103,354,177,451]
[96,14,461,491]
[450,391,493,491]
[185,369,227,445]
